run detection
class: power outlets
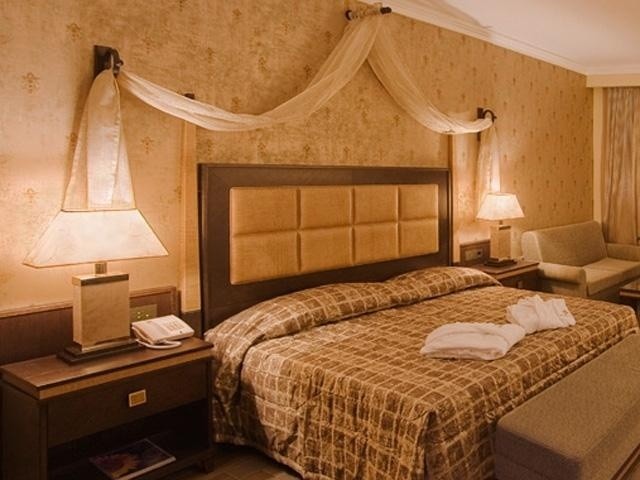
[466,248,483,261]
[130,304,158,322]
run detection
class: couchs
[519,219,640,302]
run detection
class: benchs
[494,334,640,480]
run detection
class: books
[89,437,177,480]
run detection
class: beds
[197,160,640,480]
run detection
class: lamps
[475,192,525,268]
[21,207,169,366]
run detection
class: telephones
[132,314,195,346]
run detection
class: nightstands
[469,257,540,290]
[0,336,217,480]
